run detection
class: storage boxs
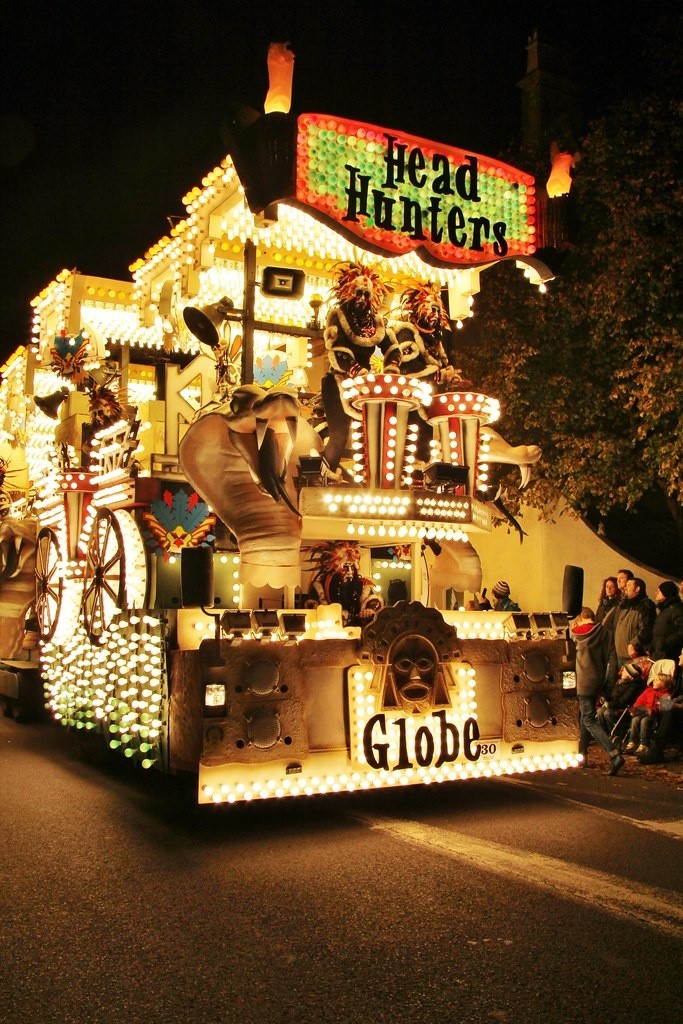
[54,390,190,471]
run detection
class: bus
[0,42,587,814]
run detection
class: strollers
[616,659,676,764]
[595,657,654,750]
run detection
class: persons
[478,582,521,612]
[322,273,402,485]
[303,544,383,628]
[569,569,683,775]
[81,398,143,475]
[394,289,471,487]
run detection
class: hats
[624,663,642,679]
[493,580,511,596]
[658,581,679,598]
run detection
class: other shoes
[633,742,649,756]
[662,743,680,762]
[601,754,625,776]
[622,740,638,755]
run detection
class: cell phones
[482,588,487,598]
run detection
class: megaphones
[33,386,69,420]
[87,360,117,388]
[260,266,306,300]
[183,296,234,346]
